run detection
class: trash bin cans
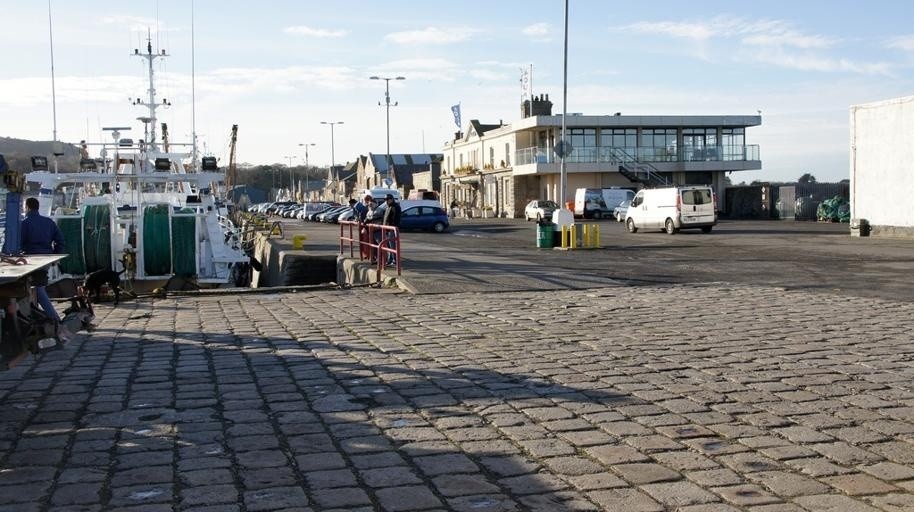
[566,201,574,211]
[536,221,556,248]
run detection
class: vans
[625,187,718,234]
[574,188,636,218]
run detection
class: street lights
[285,154,299,199]
[370,75,407,189]
[319,119,346,199]
[298,142,317,201]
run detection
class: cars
[524,200,561,222]
[249,200,449,233]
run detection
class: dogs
[77,259,126,305]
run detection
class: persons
[21,197,65,325]
[348,198,370,259]
[362,198,384,265]
[361,194,376,259]
[381,193,402,268]
